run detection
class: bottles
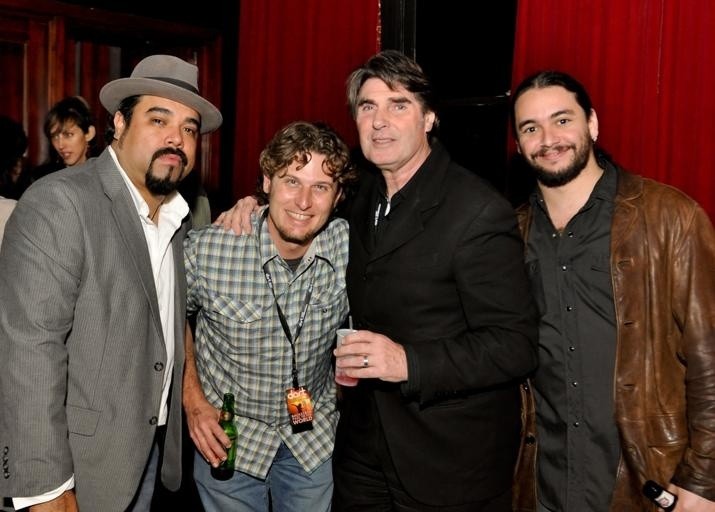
[210,393,238,481]
[644,480,678,508]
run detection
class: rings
[363,355,369,369]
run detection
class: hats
[99,54,223,135]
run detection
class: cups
[335,329,361,387]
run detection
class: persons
[183,122,352,512]
[1,55,222,512]
[215,52,538,512]
[1,116,30,239]
[510,71,714,512]
[35,96,97,182]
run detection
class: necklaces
[148,206,159,221]
